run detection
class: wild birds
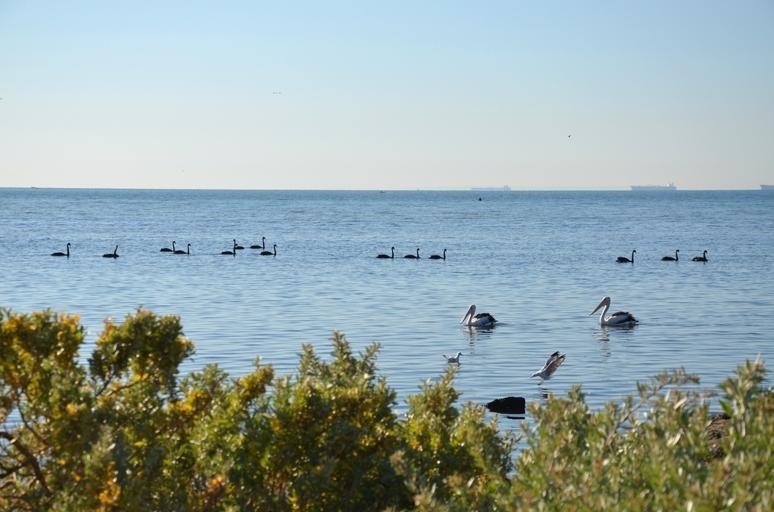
[692,249,708,262]
[218,236,277,257]
[159,240,176,252]
[616,248,637,264]
[660,249,680,262]
[102,244,119,258]
[376,246,446,260]
[174,243,191,255]
[51,242,71,257]
[529,348,565,382]
[441,351,463,365]
[459,303,498,328]
[590,296,639,327]
